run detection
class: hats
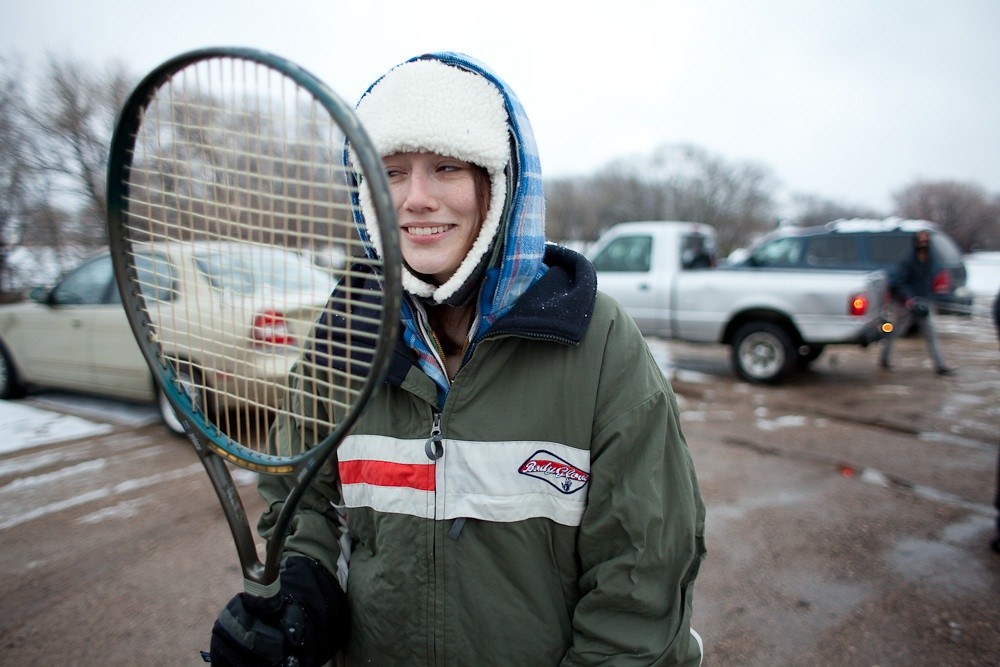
[350,56,518,307]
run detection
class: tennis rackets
[102,44,407,635]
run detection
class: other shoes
[875,356,897,373]
[936,362,957,375]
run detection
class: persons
[880,231,960,374]
[208,49,709,667]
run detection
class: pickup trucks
[580,219,895,386]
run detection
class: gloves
[210,552,351,667]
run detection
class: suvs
[718,215,977,337]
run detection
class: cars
[0,236,347,439]
